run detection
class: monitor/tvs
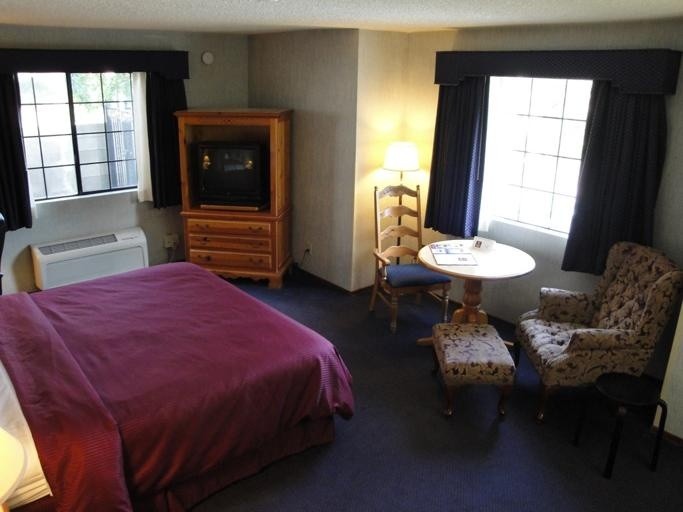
[199,143,263,205]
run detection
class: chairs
[513,237,682,427]
[365,186,451,335]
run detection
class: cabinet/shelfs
[173,105,295,292]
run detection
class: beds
[0,258,358,511]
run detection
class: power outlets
[161,232,179,250]
[304,239,313,255]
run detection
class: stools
[430,323,522,423]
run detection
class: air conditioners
[28,226,150,293]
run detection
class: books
[429,242,478,266]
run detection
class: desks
[416,237,540,355]
[568,371,670,481]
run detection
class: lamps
[381,139,421,266]
